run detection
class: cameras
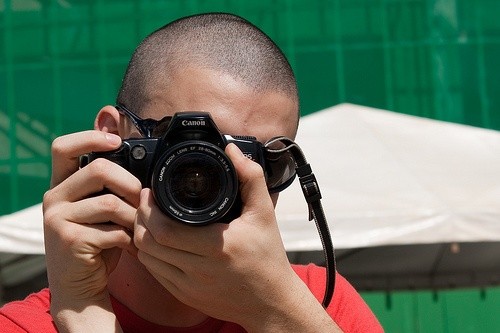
[80,111,269,228]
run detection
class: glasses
[117,103,294,193]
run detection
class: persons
[0,11,386,333]
